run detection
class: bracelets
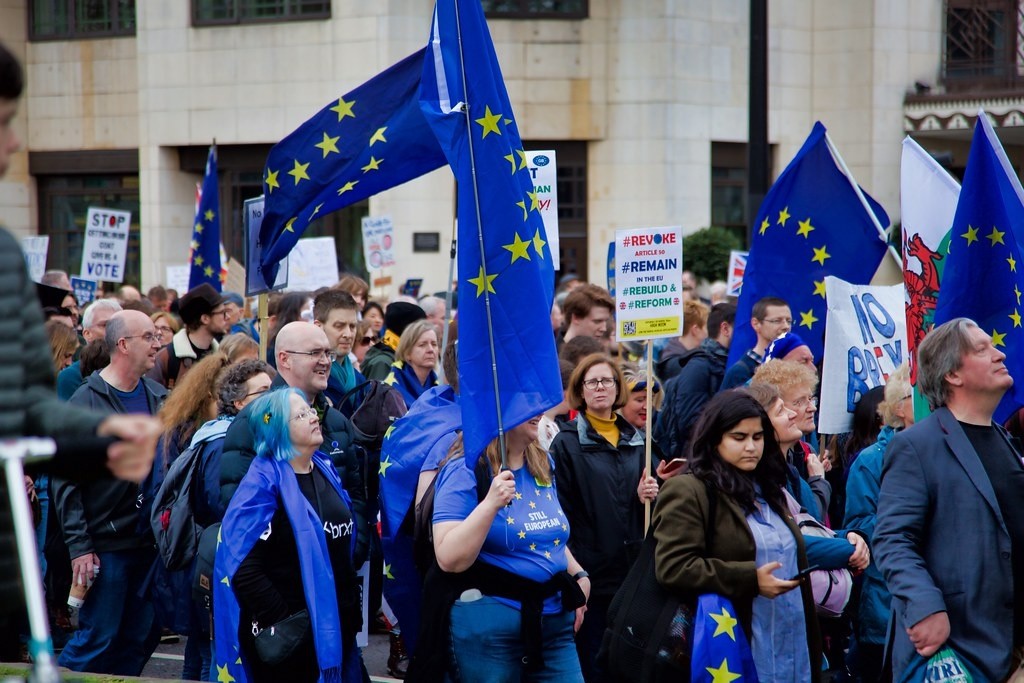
[573,571,589,581]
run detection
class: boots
[387,628,410,678]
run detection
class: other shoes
[159,627,180,644]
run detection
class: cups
[67,564,100,609]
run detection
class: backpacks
[596,529,683,676]
[337,379,407,448]
[781,487,853,619]
[652,374,679,459]
[150,434,206,572]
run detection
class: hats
[761,332,806,363]
[218,291,244,307]
[175,283,229,325]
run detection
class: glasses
[286,348,338,361]
[784,397,819,407]
[582,377,618,389]
[758,317,793,325]
[899,394,912,400]
[288,408,317,423]
[210,309,227,319]
[154,326,173,333]
[247,389,268,396]
[115,334,161,346]
[355,336,378,346]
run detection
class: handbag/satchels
[252,609,308,665]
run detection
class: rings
[650,489,651,493]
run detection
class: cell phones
[661,457,687,474]
[787,563,820,581]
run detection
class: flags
[818,276,911,435]
[188,146,221,294]
[414,0,565,471]
[900,138,962,424]
[933,110,1024,427]
[258,47,450,289]
[725,122,891,369]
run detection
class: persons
[0,40,56,663]
[32,271,1024,683]
